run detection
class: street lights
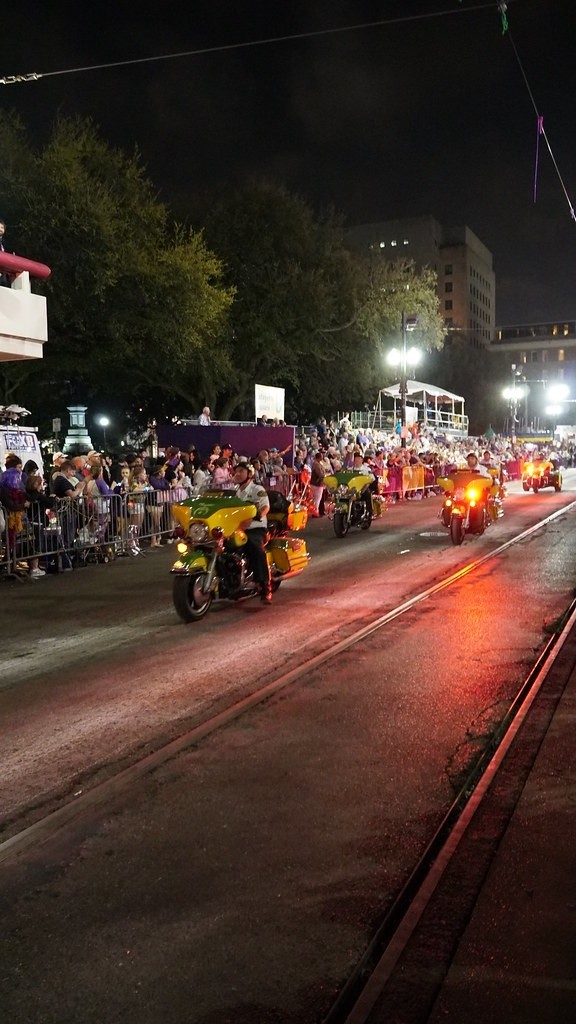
[546,384,569,440]
[386,310,422,449]
[100,418,108,452]
[503,364,524,454]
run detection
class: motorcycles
[521,461,562,494]
[436,468,508,545]
[170,489,311,622]
[322,468,388,537]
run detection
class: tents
[379,380,465,432]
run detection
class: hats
[52,452,68,461]
[88,451,102,459]
[222,443,233,450]
[152,465,167,475]
[6,454,22,468]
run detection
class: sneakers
[261,588,272,604]
[30,568,46,576]
[138,548,146,554]
[167,536,180,544]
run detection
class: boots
[151,535,164,548]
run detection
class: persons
[233,461,273,604]
[0,407,576,578]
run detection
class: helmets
[233,462,255,478]
